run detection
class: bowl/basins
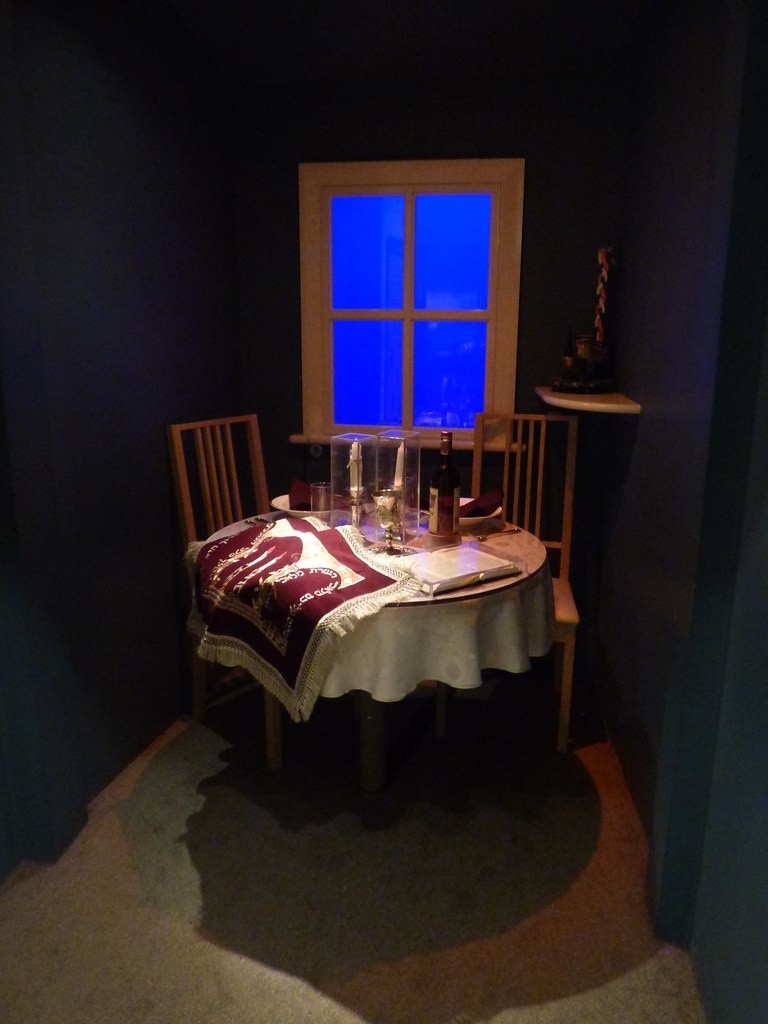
[460,498,505,528]
[272,493,342,519]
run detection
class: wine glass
[371,489,400,556]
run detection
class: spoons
[473,528,521,541]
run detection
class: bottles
[429,430,460,537]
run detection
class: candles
[394,442,406,485]
[350,438,362,489]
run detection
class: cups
[310,482,331,513]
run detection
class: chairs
[437,411,579,751]
[166,414,282,771]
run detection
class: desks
[200,508,556,791]
